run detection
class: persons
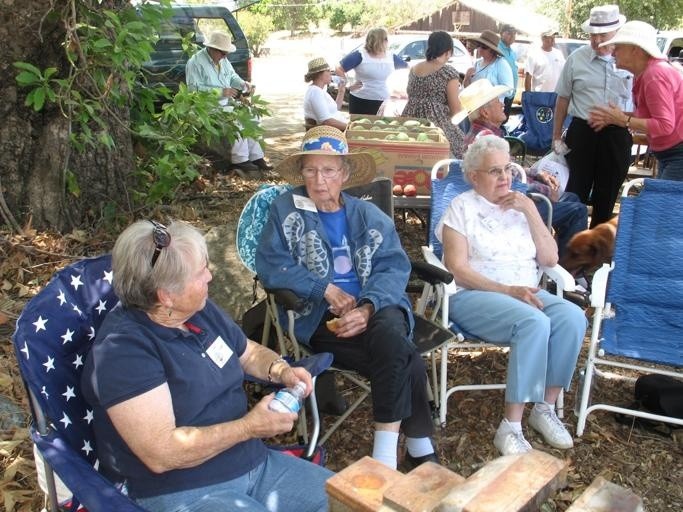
[586,18,683,182]
[428,134,590,456]
[81,216,338,512]
[549,3,635,230]
[304,23,566,154]
[185,31,274,172]
[253,124,442,475]
[450,78,589,298]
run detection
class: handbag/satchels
[635,374,683,428]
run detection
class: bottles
[266,374,310,419]
[329,76,346,87]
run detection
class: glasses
[149,218,172,268]
[476,164,515,178]
[476,44,487,49]
[298,160,344,179]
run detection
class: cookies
[326,318,340,333]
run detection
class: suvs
[129,5,255,112]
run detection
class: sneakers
[493,427,534,455]
[404,446,439,471]
[528,403,575,450]
[254,158,274,170]
[231,160,259,172]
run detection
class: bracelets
[266,357,289,382]
[625,115,632,128]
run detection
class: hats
[304,56,333,83]
[580,5,627,34]
[276,125,378,192]
[501,23,518,33]
[464,29,506,58]
[201,32,237,54]
[598,21,668,60]
[540,29,560,37]
[451,78,510,126]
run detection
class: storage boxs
[342,115,452,196]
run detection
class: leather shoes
[548,279,589,306]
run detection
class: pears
[350,119,434,141]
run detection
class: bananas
[356,148,388,164]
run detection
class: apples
[404,184,416,196]
[392,186,402,195]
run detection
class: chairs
[503,136,527,164]
[576,178,683,438]
[415,156,577,432]
[241,178,453,447]
[520,91,560,152]
[11,254,322,512]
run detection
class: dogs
[557,214,620,280]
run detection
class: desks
[393,195,432,228]
